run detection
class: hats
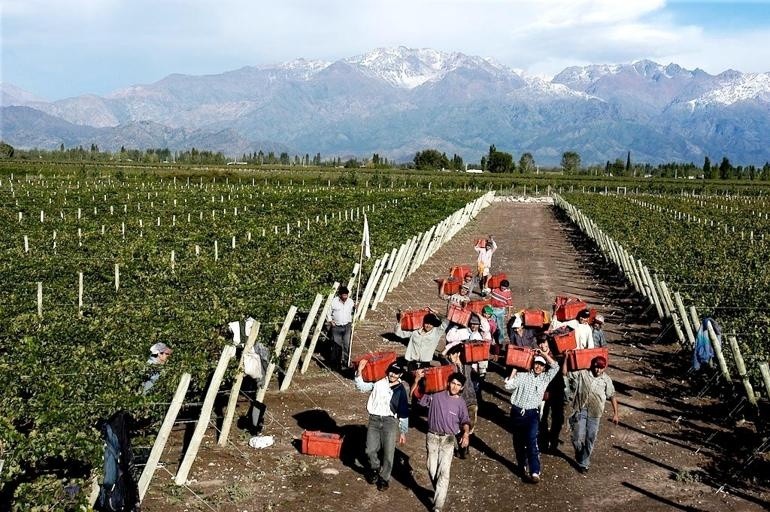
[469,315,481,325]
[593,314,606,324]
[533,356,547,365]
[384,360,404,374]
[441,339,464,356]
[338,286,350,294]
[590,356,607,370]
[499,279,510,286]
[482,305,494,315]
[148,342,168,357]
[577,309,590,319]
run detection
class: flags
[359,214,373,261]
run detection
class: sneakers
[518,464,541,483]
[366,468,379,484]
[376,476,389,491]
[452,446,472,460]
[575,464,589,475]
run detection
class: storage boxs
[446,300,492,328]
[410,363,457,394]
[485,273,507,289]
[438,278,461,295]
[351,351,397,383]
[574,347,608,370]
[396,308,429,331]
[476,238,486,248]
[504,343,535,372]
[523,310,545,328]
[462,339,491,364]
[551,325,577,353]
[552,298,598,324]
[301,430,344,458]
[450,265,471,282]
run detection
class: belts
[427,429,446,437]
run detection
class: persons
[438,278,472,316]
[535,331,564,452]
[507,309,550,348]
[473,234,497,294]
[326,286,359,368]
[393,306,449,383]
[551,302,594,370]
[450,262,485,303]
[482,274,513,341]
[354,358,409,492]
[577,298,608,348]
[409,368,473,512]
[446,313,493,407]
[562,348,619,474]
[504,349,561,482]
[480,306,501,364]
[139,342,173,395]
